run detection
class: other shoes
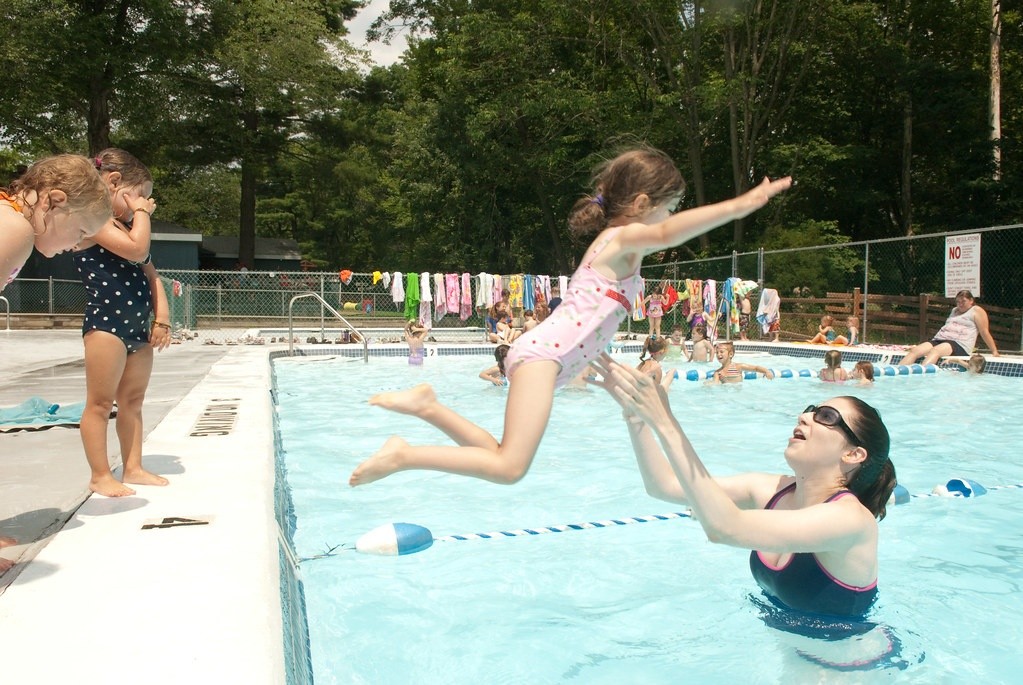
[171,331,437,345]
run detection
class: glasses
[803,405,862,447]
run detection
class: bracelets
[152,320,171,333]
[134,208,151,216]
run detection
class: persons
[0,153,111,294]
[349,144,792,487]
[806,316,859,346]
[940,354,986,373]
[73,147,171,497]
[479,288,597,386]
[820,350,874,384]
[582,351,895,614]
[899,290,1000,366]
[638,275,780,386]
[404,319,427,364]
